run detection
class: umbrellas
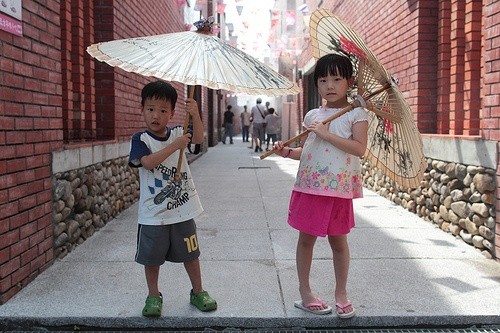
[260,8,428,188]
[87,16,300,182]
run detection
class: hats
[257,98,262,102]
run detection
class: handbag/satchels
[263,123,267,128]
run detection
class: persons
[127,81,217,317]
[221,98,279,152]
[272,54,368,318]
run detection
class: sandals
[189,289,217,312]
[142,292,163,316]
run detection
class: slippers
[294,297,332,314]
[336,300,355,318]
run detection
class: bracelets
[283,148,292,158]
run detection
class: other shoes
[260,148,263,151]
[255,145,259,152]
